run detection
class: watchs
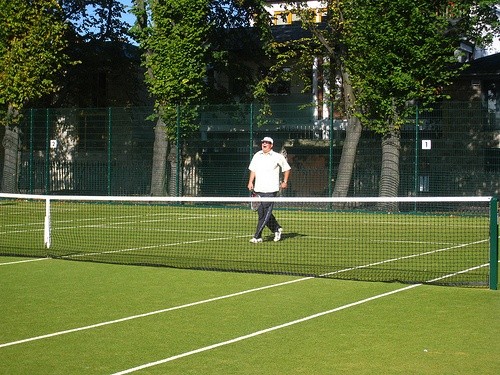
[283,181,288,184]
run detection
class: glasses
[261,142,271,144]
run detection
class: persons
[247,136,291,244]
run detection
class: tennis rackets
[250,189,261,212]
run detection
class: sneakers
[249,237,262,243]
[274,228,283,242]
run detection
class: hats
[261,137,273,144]
